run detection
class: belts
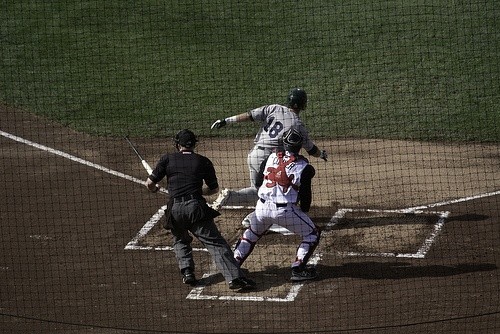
[175,194,200,202]
[259,198,287,208]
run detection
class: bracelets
[152,187,158,193]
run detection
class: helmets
[283,128,304,147]
[287,89,307,106]
[173,129,196,149]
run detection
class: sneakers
[290,269,318,279]
[230,277,256,289]
[183,274,195,284]
[211,188,230,212]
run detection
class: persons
[232,128,320,281]
[146,128,255,289]
[211,88,328,230]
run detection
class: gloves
[211,117,227,131]
[318,149,328,162]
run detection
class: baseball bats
[125,136,161,187]
